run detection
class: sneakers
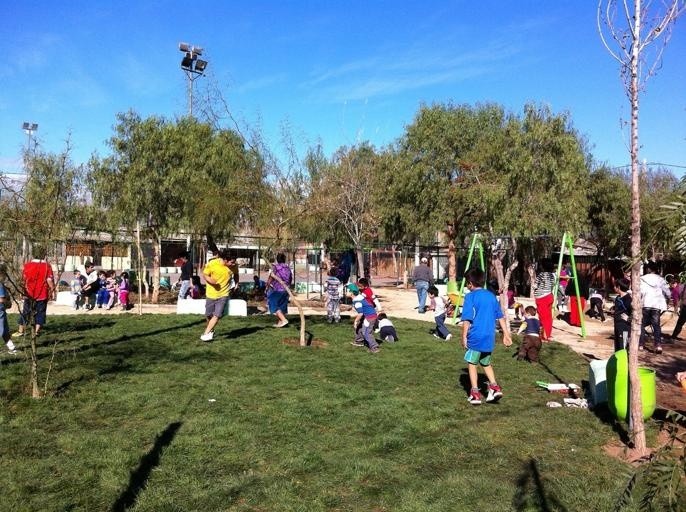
[466,388,483,406]
[200,328,215,341]
[12,331,25,337]
[272,317,288,328]
[71,304,126,311]
[350,341,380,355]
[485,384,503,403]
[8,346,21,354]
[639,346,663,354]
[445,334,452,342]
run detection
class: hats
[345,283,359,293]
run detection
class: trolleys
[444,291,466,320]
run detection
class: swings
[488,238,521,309]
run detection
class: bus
[204,243,282,279]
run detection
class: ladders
[451,232,486,327]
[551,230,586,339]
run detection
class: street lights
[177,38,210,127]
[22,118,44,162]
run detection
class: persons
[11,245,56,337]
[0,263,21,354]
[174,251,292,342]
[374,312,398,343]
[459,266,512,406]
[359,278,382,330]
[323,266,341,324]
[345,284,380,353]
[426,286,452,341]
[70,260,131,313]
[412,257,434,314]
[507,256,686,366]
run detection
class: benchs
[177,299,248,317]
[56,290,116,308]
